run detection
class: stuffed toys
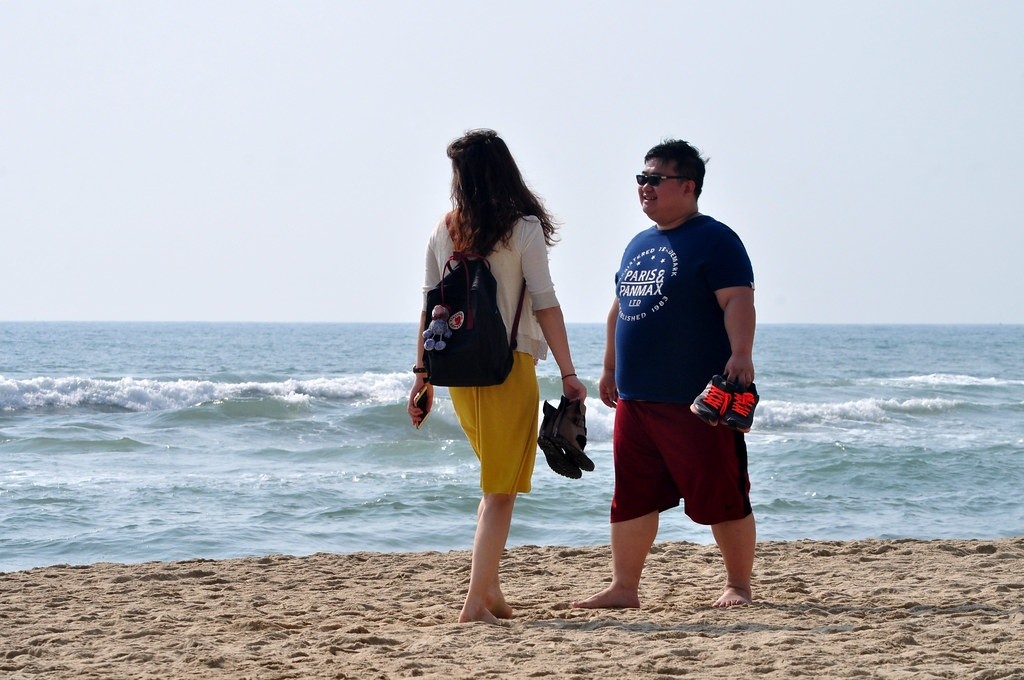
[421,305,452,351]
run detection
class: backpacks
[424,213,527,387]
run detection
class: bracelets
[562,374,577,379]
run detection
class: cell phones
[413,386,429,429]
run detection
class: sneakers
[690,373,736,427]
[719,382,760,434]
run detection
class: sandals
[550,395,596,472]
[537,399,582,479]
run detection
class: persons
[408,128,586,623]
[572,138,756,608]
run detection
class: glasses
[636,174,690,186]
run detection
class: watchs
[413,364,427,373]
